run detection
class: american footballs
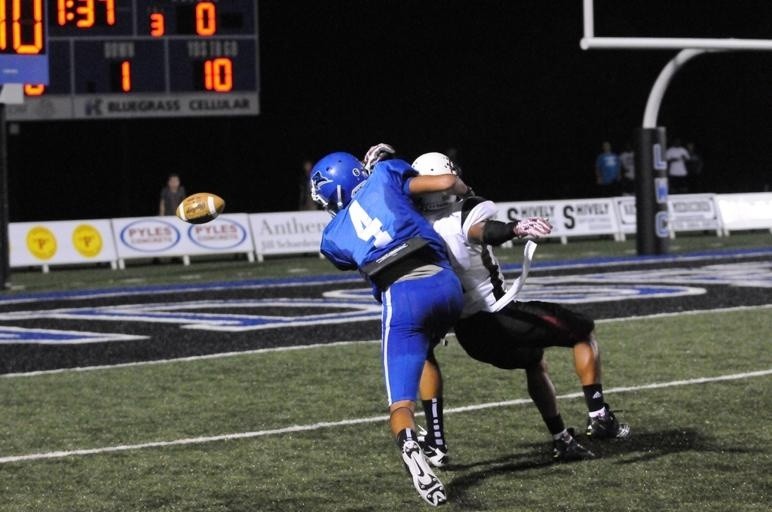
[177,193,225,224]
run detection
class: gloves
[513,216,556,243]
[360,141,395,175]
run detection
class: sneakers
[586,402,633,444]
[552,426,610,462]
[399,437,448,509]
[416,425,449,468]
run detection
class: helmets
[406,150,465,213]
[305,151,370,222]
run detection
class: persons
[590,131,697,196]
[158,173,187,217]
[309,150,478,506]
[409,150,631,462]
[297,159,320,210]
